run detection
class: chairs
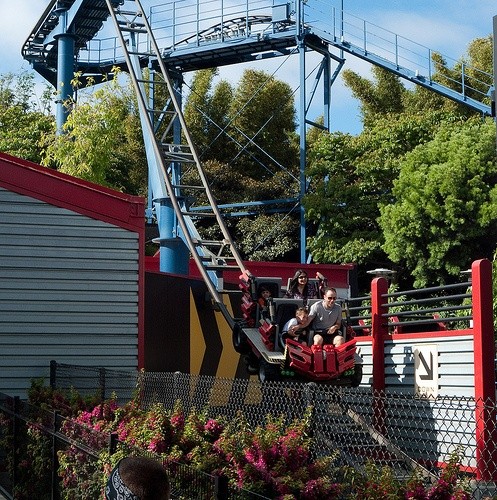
[253,277,281,326]
[288,278,321,299]
[272,298,305,350]
[306,300,346,346]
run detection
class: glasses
[327,297,337,301]
[298,275,308,279]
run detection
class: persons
[104,455,172,500]
[257,285,273,311]
[283,269,330,307]
[278,305,307,353]
[290,287,346,365]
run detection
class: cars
[233,276,365,388]
